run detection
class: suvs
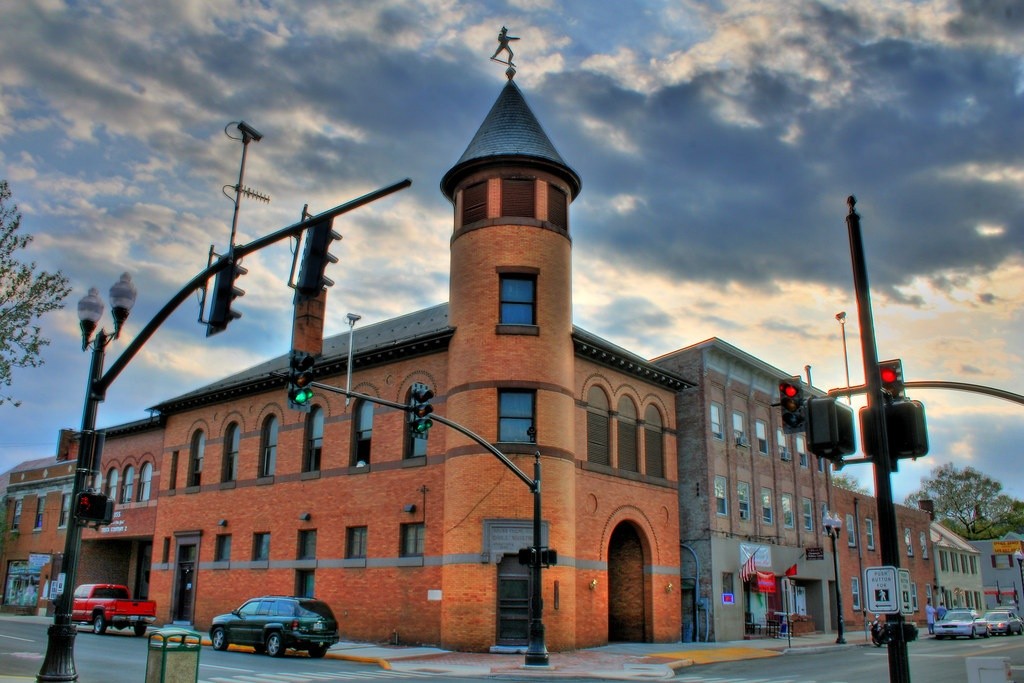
[209,594,340,658]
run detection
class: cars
[983,611,1023,636]
[933,609,989,639]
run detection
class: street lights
[35,271,139,683]
[822,512,847,643]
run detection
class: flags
[740,554,757,582]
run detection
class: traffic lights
[781,375,805,434]
[409,383,433,440]
[287,348,316,413]
[878,360,904,400]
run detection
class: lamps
[480,552,489,564]
[404,504,417,512]
[217,518,227,527]
[590,578,598,590]
[665,582,672,592]
[300,512,310,521]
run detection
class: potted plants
[790,613,818,637]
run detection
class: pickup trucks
[72,583,157,637]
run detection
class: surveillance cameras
[237,120,264,142]
[835,311,847,320]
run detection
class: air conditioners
[736,436,749,446]
[781,450,792,461]
[739,509,748,518]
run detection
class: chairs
[765,610,780,638]
[745,612,761,636]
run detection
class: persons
[491,27,520,66]
[935,602,947,620]
[926,600,936,635]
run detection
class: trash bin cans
[144,628,202,681]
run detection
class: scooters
[868,614,888,646]
